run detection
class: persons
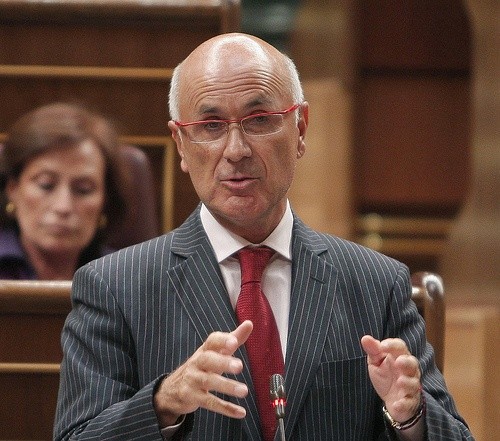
[0,103,142,281]
[52,33,477,441]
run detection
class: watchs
[382,390,427,430]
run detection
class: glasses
[174,105,297,143]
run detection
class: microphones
[269,374,288,441]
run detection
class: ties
[234,247,285,440]
